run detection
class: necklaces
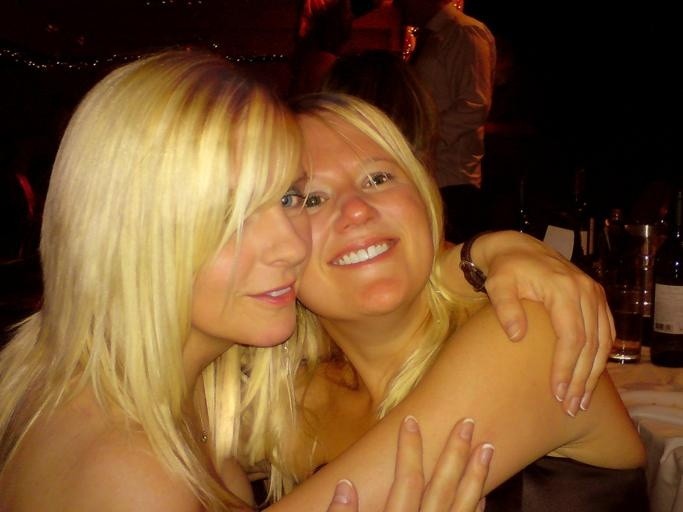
[196,396,210,444]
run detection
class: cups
[603,271,643,364]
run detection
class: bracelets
[458,231,488,294]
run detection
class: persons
[400,1,498,245]
[1,46,619,512]
[320,46,442,178]
[250,90,650,512]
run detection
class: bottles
[651,188,683,368]
